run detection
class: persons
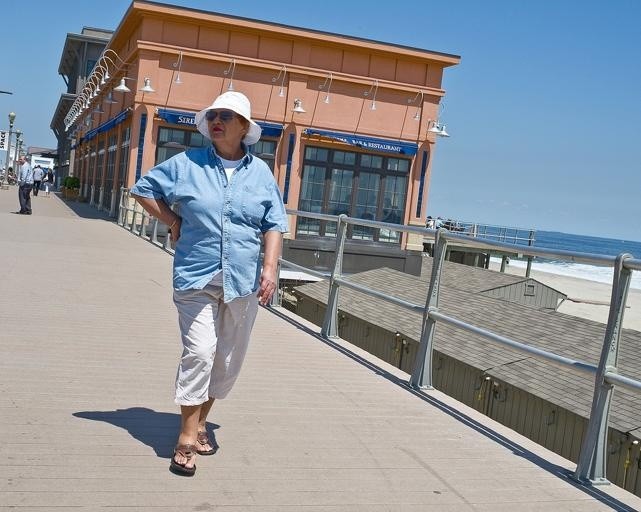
[128,90,293,476]
[33,165,54,196]
[16,156,34,215]
[427,216,465,231]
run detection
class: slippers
[197,431,217,454]
[171,445,197,475]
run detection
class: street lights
[3,113,23,184]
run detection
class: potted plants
[61,176,80,201]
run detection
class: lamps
[272,65,287,97]
[319,71,333,103]
[290,98,307,113]
[407,90,423,120]
[63,49,157,132]
[172,50,184,84]
[364,78,379,111]
[224,56,236,91]
[428,103,451,138]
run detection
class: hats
[196,92,262,147]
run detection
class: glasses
[204,111,240,122]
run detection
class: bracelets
[169,215,180,229]
